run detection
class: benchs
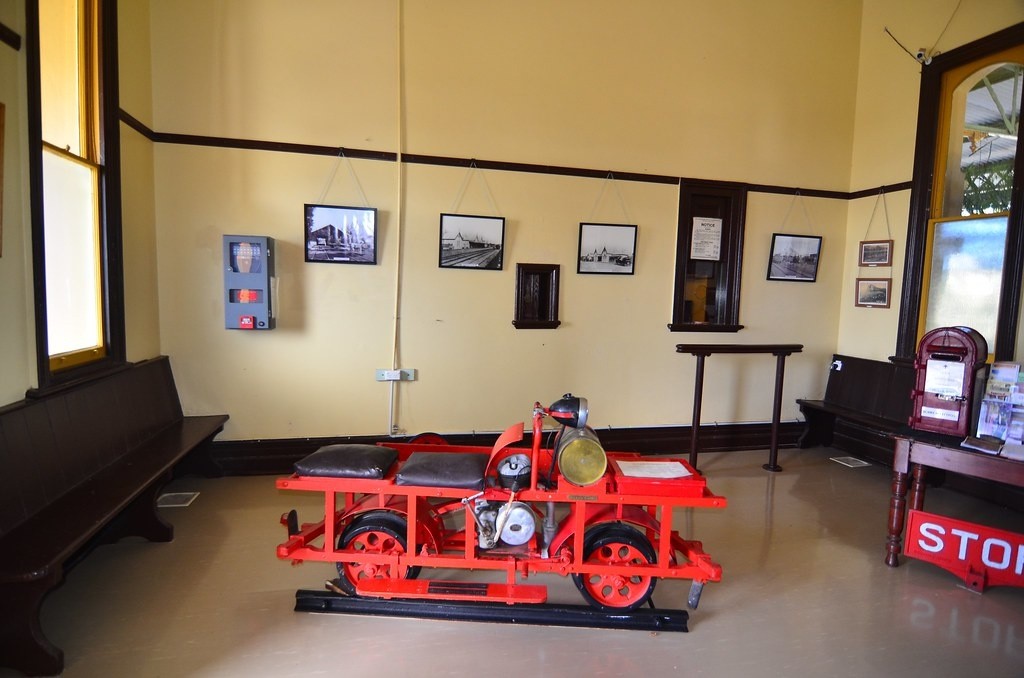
[0,355,229,677]
[795,354,917,449]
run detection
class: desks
[885,425,1024,569]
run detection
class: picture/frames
[304,203,377,265]
[858,240,894,266]
[439,212,506,271]
[766,233,822,282]
[577,222,638,275]
[855,277,892,308]
